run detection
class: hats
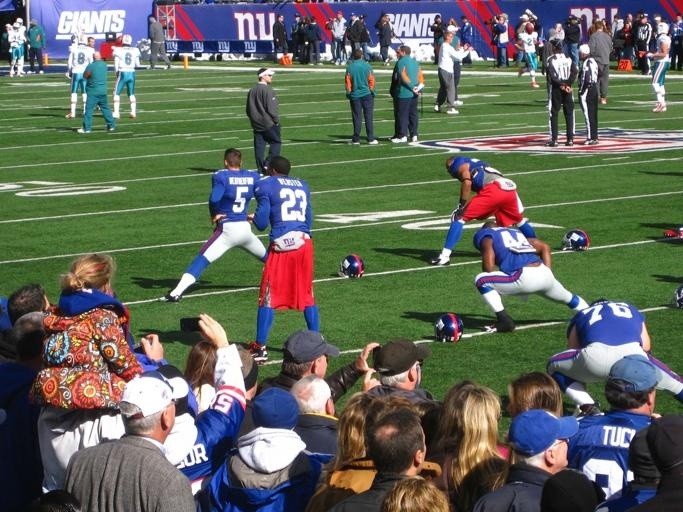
[550,39,563,49]
[120,376,189,419]
[252,388,298,430]
[283,332,339,363]
[509,356,682,507]
[374,340,430,376]
[580,44,590,54]
[258,69,274,78]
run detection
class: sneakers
[77,128,90,134]
[346,134,419,144]
[112,112,119,118]
[432,99,465,114]
[479,319,515,333]
[247,341,268,364]
[650,101,670,113]
[430,255,450,265]
[65,114,75,119]
[530,82,539,87]
[544,139,601,147]
[601,98,607,104]
[130,112,136,117]
[158,292,179,302]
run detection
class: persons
[158,147,271,303]
[244,67,284,172]
[3,18,46,76]
[1,249,683,511]
[149,14,171,71]
[472,218,590,334]
[546,38,602,146]
[638,22,671,112]
[373,11,395,66]
[433,30,472,115]
[65,30,142,134]
[245,154,319,366]
[490,8,682,76]
[273,9,371,66]
[514,22,539,88]
[345,49,379,145]
[428,157,535,269]
[431,15,473,66]
[389,46,425,143]
[588,20,613,104]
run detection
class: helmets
[122,34,132,45]
[526,23,534,32]
[78,34,87,44]
[658,23,668,34]
[338,254,365,278]
[434,313,463,343]
[563,230,590,251]
[672,286,683,309]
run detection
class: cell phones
[180,318,202,333]
[373,347,382,371]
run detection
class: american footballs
[526,23,534,34]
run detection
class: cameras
[132,341,151,354]
